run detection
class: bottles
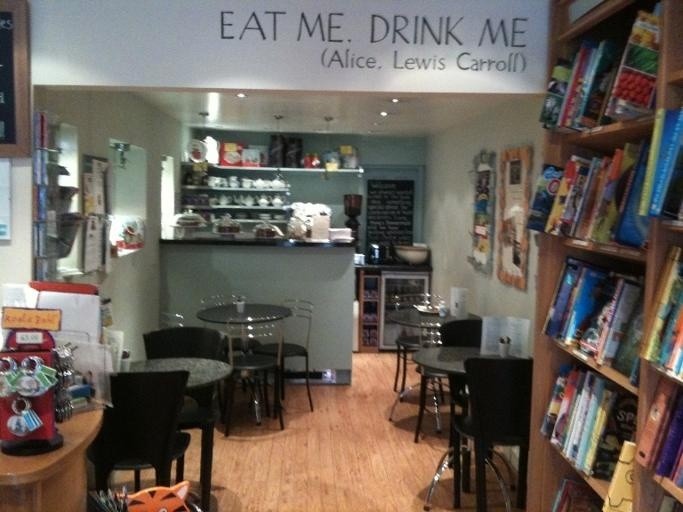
[304,152,321,168]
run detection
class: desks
[0,396,107,512]
[388,307,483,433]
[129,356,234,512]
[411,346,522,511]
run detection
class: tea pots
[208,194,286,206]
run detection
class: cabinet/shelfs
[180,165,366,234]
[356,264,434,354]
[527,0,682,512]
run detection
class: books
[528,2,683,512]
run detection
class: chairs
[86,369,190,495]
[454,356,532,512]
[394,292,445,405]
[415,318,482,444]
[142,291,315,437]
